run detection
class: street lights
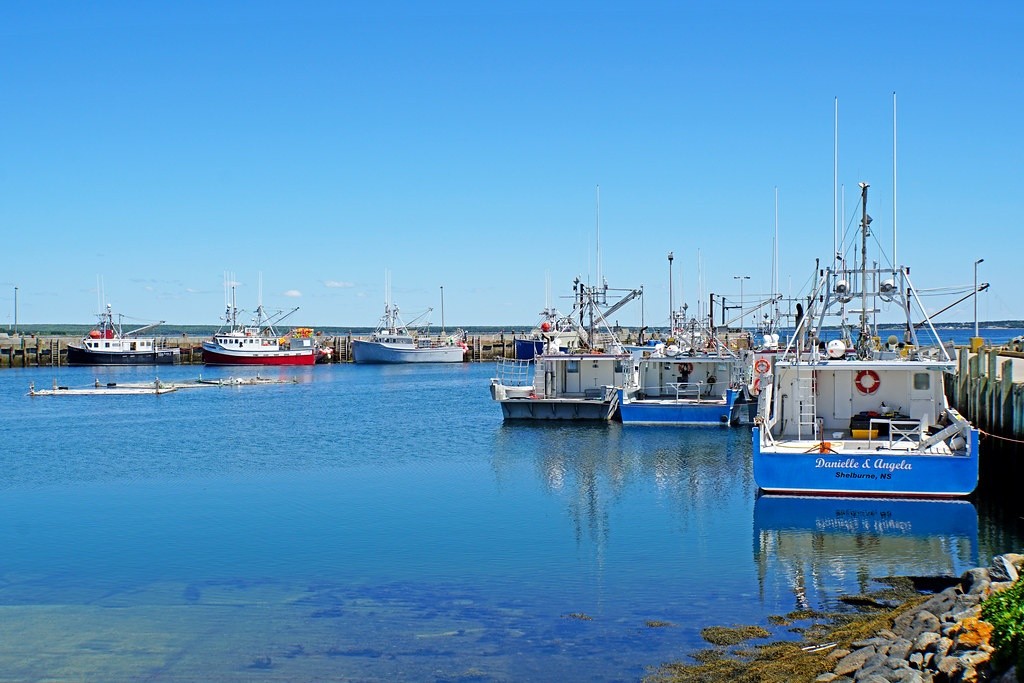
[972,258,986,338]
[440,285,444,333]
[15,287,20,334]
[734,276,752,328]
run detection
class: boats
[751,91,987,497]
[747,489,982,614]
[64,273,181,365]
[490,185,671,422]
[617,248,764,427]
[351,262,464,363]
[202,269,319,365]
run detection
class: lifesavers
[754,378,770,393]
[754,359,770,374]
[678,363,693,375]
[855,370,880,394]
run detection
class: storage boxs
[852,430,878,439]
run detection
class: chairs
[813,417,824,441]
